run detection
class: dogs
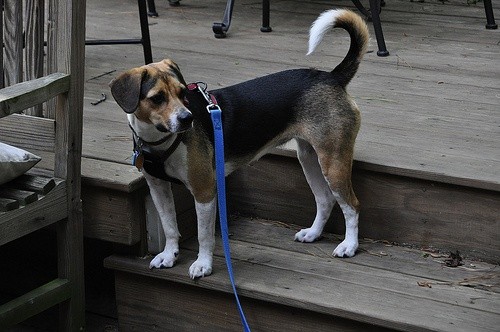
[108,7,372,281]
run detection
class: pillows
[0,141,42,185]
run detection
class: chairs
[0,1,85,332]
